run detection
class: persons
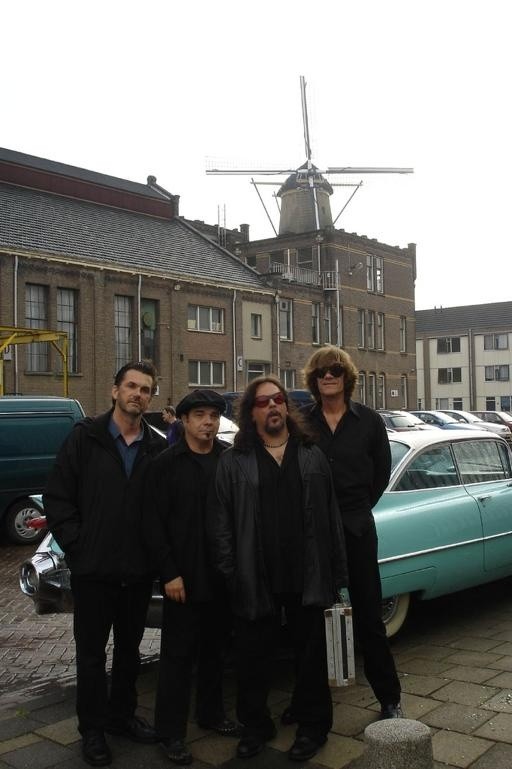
[128,388,243,767]
[204,375,347,762]
[160,406,186,445]
[280,344,403,726]
[43,359,171,768]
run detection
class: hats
[175,390,226,417]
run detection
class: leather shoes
[156,728,194,766]
[198,712,242,737]
[236,719,277,756]
[105,715,159,744]
[282,704,300,726]
[379,701,403,720]
[82,728,111,768]
[289,734,329,759]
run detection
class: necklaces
[264,437,288,448]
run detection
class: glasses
[253,391,285,408]
[313,366,345,379]
